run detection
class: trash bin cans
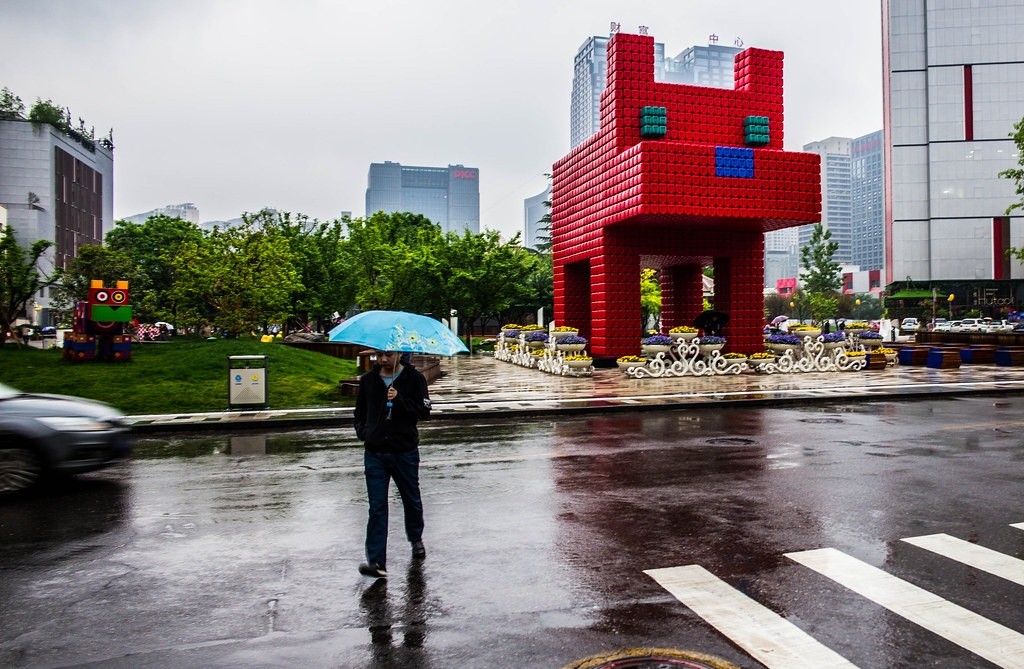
[227,355,269,410]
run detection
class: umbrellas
[155,322,174,330]
[694,309,729,329]
[326,310,470,420]
[771,316,789,324]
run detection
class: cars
[41,326,57,335]
[935,317,1024,334]
[784,318,881,327]
[900,317,920,330]
[0,382,134,503]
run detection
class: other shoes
[412,539,425,558]
[359,562,387,576]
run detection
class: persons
[157,324,169,341]
[839,321,845,330]
[353,348,433,578]
[704,315,723,338]
[824,322,830,334]
[778,320,789,335]
